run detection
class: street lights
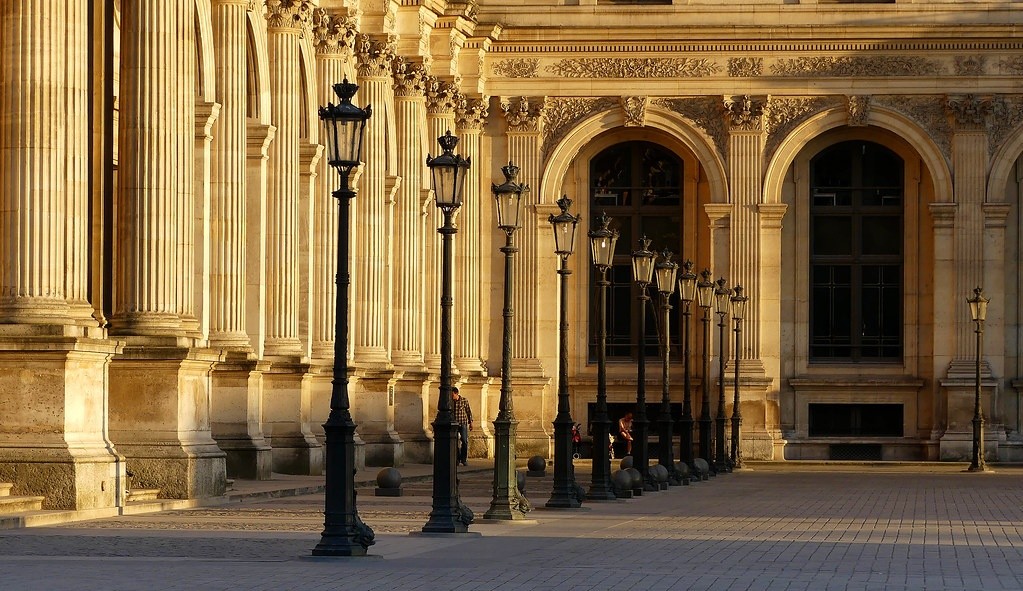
[965,286,994,470]
[311,71,381,557]
[625,234,660,493]
[695,266,718,479]
[545,194,585,509]
[729,282,751,469]
[654,244,684,487]
[587,207,626,501]
[713,277,732,474]
[677,257,701,482]
[424,129,473,532]
[481,159,533,523]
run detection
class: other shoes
[460,460,468,466]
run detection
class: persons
[452,386,473,467]
[619,412,634,455]
